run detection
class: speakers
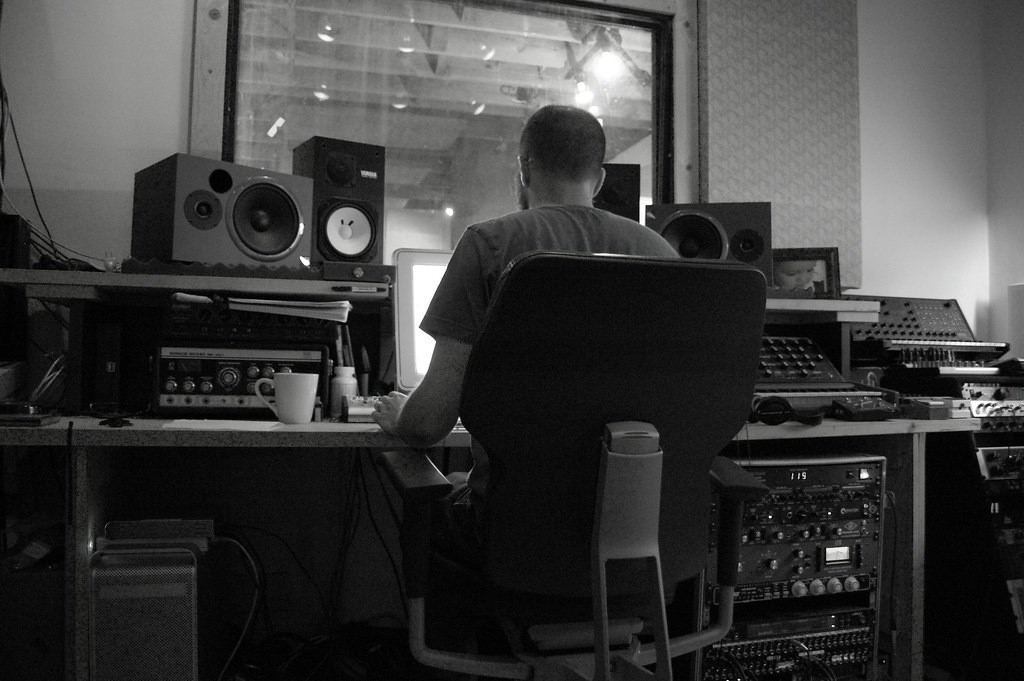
[593,163,772,288]
[87,543,212,681]
[131,135,385,270]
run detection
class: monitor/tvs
[392,248,454,395]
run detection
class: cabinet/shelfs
[0,266,982,681]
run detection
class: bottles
[331,366,357,419]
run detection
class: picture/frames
[772,247,841,301]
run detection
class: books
[228,297,353,324]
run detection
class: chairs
[376,251,767,681]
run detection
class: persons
[774,259,824,295]
[370,104,683,681]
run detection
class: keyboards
[342,394,462,426]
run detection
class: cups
[255,373,319,424]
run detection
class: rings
[376,402,383,411]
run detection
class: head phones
[747,395,824,426]
